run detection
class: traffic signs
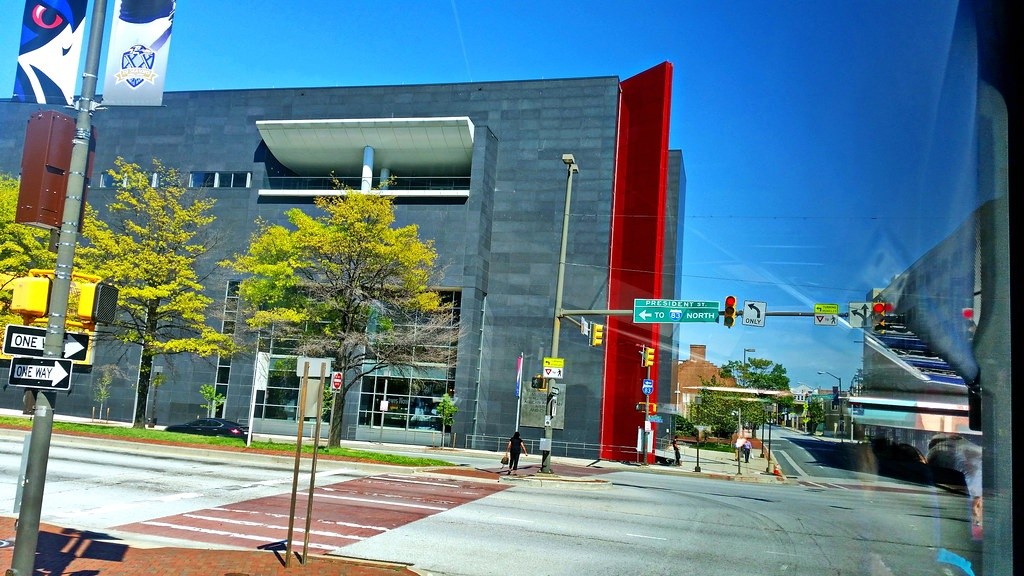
[814,303,838,314]
[8,356,73,390]
[633,298,720,324]
[741,300,767,327]
[2,324,90,362]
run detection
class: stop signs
[332,372,343,389]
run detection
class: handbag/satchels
[750,451,754,460]
[500,455,508,465]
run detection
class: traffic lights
[644,347,655,367]
[872,302,893,335]
[531,377,546,389]
[592,324,604,347]
[724,295,736,327]
[78,283,119,324]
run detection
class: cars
[164,417,253,444]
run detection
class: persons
[736,436,752,463]
[673,436,683,466]
[506,431,528,474]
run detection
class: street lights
[538,153,581,474]
[743,348,755,387]
[817,371,844,444]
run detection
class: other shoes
[512,473,516,476]
[678,464,682,466]
[508,471,511,475]
[744,461,748,463]
[747,461,749,463]
[675,462,678,465]
